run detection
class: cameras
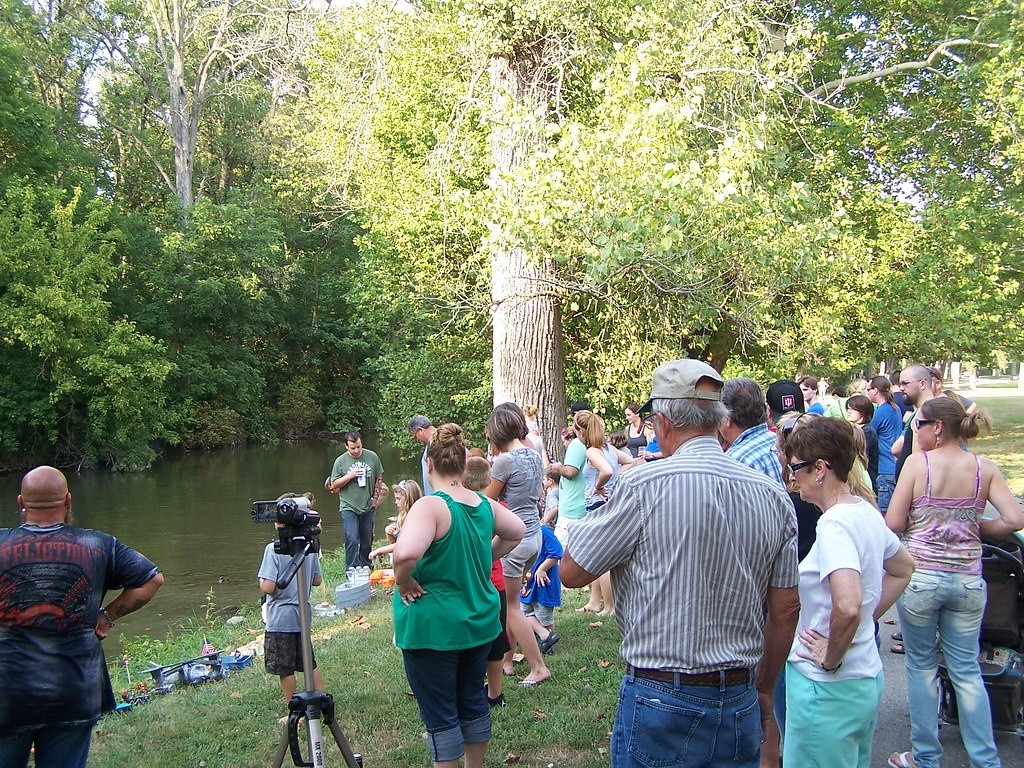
[251,498,322,555]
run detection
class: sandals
[891,643,905,653]
[892,632,904,640]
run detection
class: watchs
[100,606,115,628]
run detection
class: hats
[635,358,724,414]
[765,380,805,422]
[568,402,589,414]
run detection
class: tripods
[273,560,361,768]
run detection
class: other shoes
[503,668,515,676]
[540,634,558,652]
[484,682,505,707]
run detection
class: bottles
[348,566,370,587]
[1007,657,1021,675]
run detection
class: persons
[255,492,326,726]
[881,395,1024,768]
[331,431,384,582]
[558,359,801,768]
[0,465,164,767]
[390,422,526,768]
[324,365,1024,768]
[780,414,916,768]
[571,410,637,617]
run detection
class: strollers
[933,517,1024,743]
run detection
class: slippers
[887,752,912,768]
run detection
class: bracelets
[594,483,604,490]
[820,654,843,674]
[650,453,656,459]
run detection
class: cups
[357,468,366,486]
[639,447,645,459]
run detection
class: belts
[626,663,754,687]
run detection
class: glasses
[916,419,939,429]
[783,411,808,442]
[902,378,928,387]
[787,460,831,476]
